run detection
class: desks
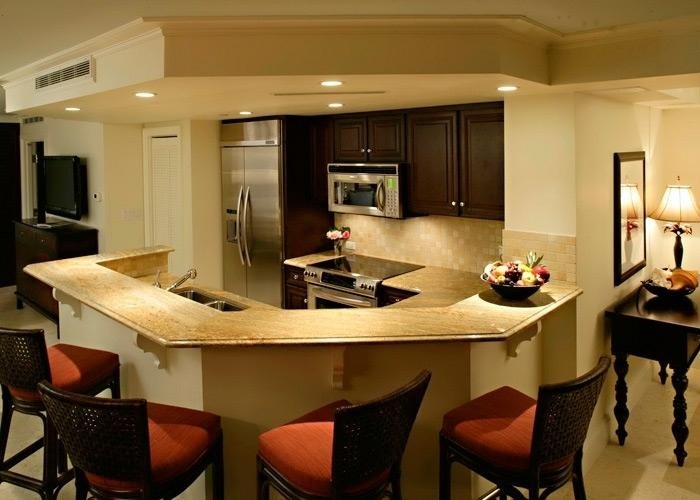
[606,268,700,466]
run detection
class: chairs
[256,368,432,500]
[0,328,120,500]
[37,381,224,500]
[439,354,610,500]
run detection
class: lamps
[646,176,700,268]
[621,183,644,268]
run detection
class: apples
[532,264,549,283]
[521,272,536,286]
[494,265,509,277]
[497,275,505,282]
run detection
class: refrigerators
[220,119,333,309]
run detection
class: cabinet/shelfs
[11,217,97,339]
[378,286,418,307]
[407,103,504,220]
[286,266,306,309]
[328,114,405,160]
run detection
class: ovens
[306,283,380,309]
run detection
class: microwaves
[327,163,431,220]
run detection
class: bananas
[484,264,497,282]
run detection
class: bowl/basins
[480,272,541,302]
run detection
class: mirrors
[615,152,647,286]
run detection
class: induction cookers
[303,255,424,298]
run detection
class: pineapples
[519,249,544,272]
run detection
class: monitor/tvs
[36,155,81,220]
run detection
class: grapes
[505,263,522,285]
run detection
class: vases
[333,240,344,254]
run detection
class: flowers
[325,225,351,255]
